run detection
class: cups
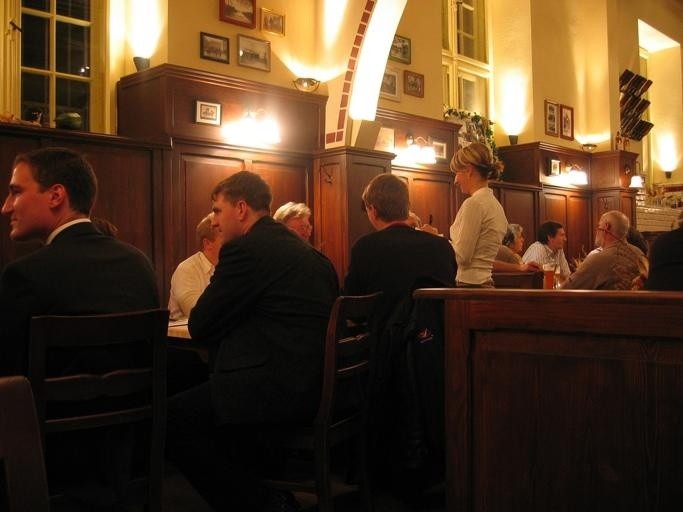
[542,264,555,291]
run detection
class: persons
[165,170,340,512]
[273,201,312,242]
[335,173,458,374]
[560,210,649,290]
[0,148,160,511]
[167,211,224,327]
[522,222,571,289]
[644,226,683,291]
[90,217,119,238]
[491,224,543,273]
[422,143,508,289]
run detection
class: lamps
[582,144,597,152]
[406,135,437,163]
[294,77,320,92]
[133,57,150,71]
[509,134,518,145]
[665,172,672,178]
[566,160,587,185]
[625,165,643,187]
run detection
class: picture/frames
[376,127,395,151]
[547,157,561,176]
[200,31,229,64]
[560,104,574,141]
[379,68,401,102]
[193,99,222,127]
[404,70,424,98]
[544,100,560,137]
[433,140,448,162]
[219,0,256,29]
[238,33,271,72]
[260,7,285,36]
[388,34,411,64]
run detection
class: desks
[167,325,208,368]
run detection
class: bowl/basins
[54,112,85,129]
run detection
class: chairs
[493,271,543,289]
[258,291,384,512]
[28,308,168,511]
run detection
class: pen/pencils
[429,214,432,225]
